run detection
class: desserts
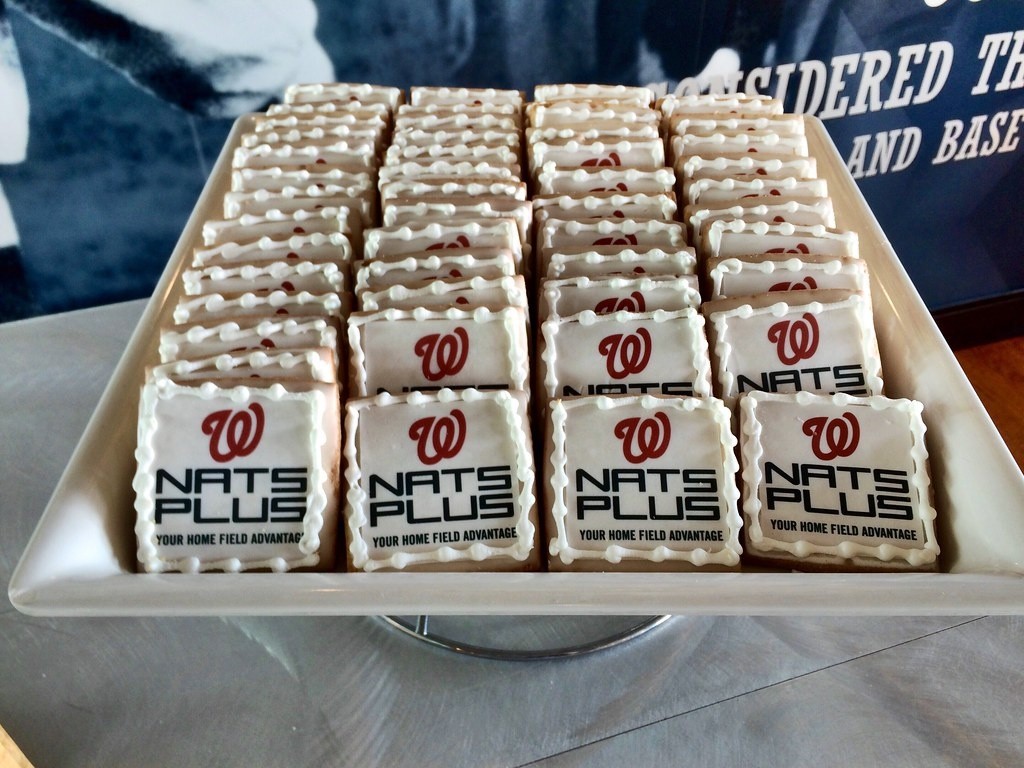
[131,80,947,576]
